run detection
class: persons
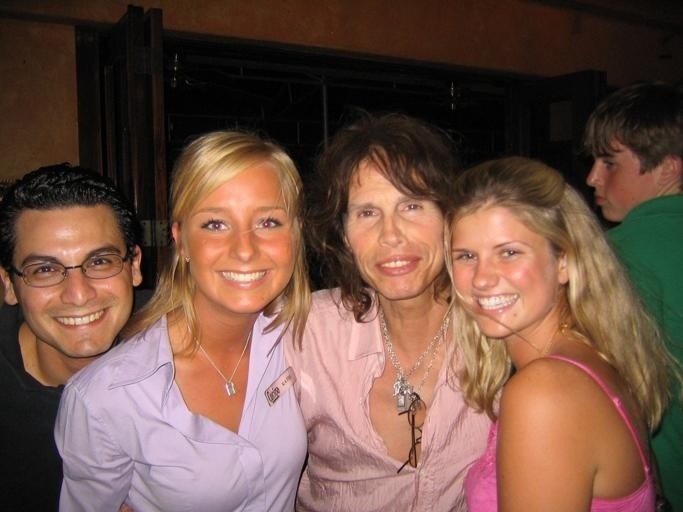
[55,125,309,511]
[1,162,143,512]
[586,79,683,512]
[442,154,682,512]
[282,111,504,512]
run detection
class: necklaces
[178,310,256,396]
[375,305,451,413]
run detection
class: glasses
[7,253,130,288]
[396,393,426,475]
